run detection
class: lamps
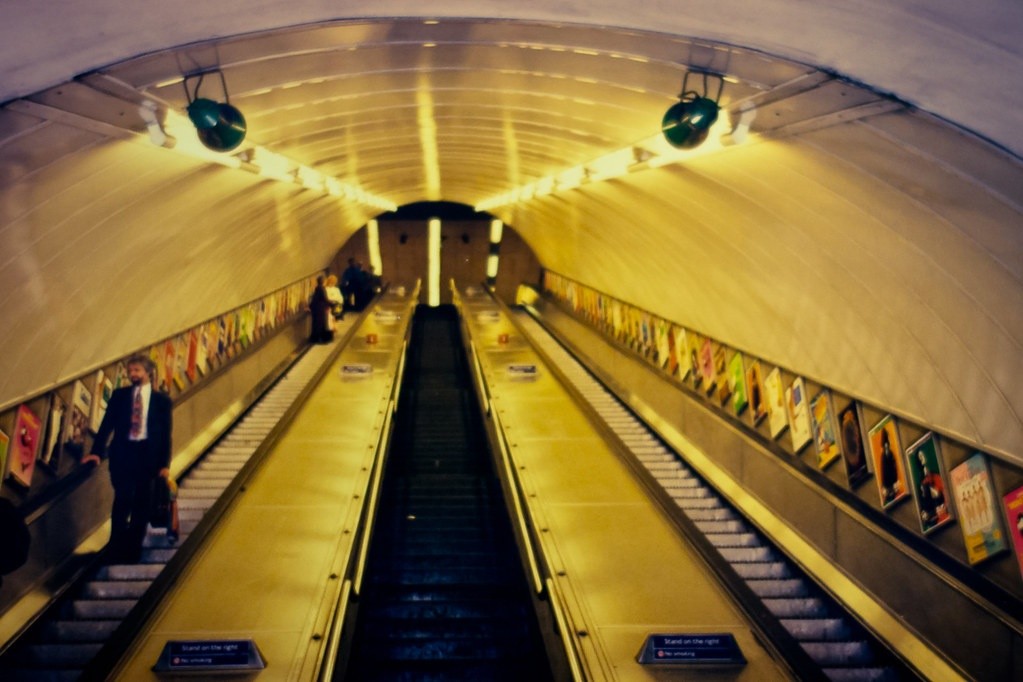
[182,70,247,153]
[661,70,725,151]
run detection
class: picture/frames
[0,272,329,494]
[543,270,1023,580]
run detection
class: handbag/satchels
[149,472,179,543]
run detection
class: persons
[916,449,948,529]
[81,358,172,564]
[879,428,898,506]
[339,257,375,306]
[309,275,343,344]
[961,481,989,529]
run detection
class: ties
[131,387,141,437]
